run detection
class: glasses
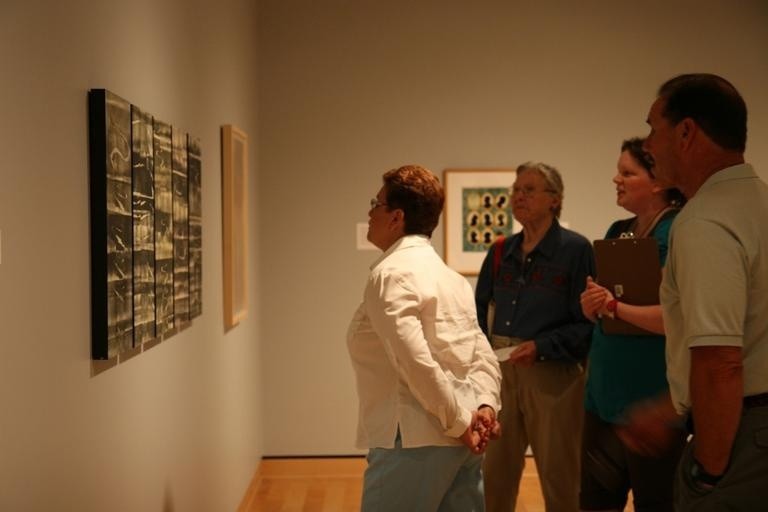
[370,198,389,210]
[508,186,555,196]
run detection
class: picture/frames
[442,168,522,277]
[222,124,248,329]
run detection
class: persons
[643,72,767,512]
[478,163,599,512]
[345,164,502,512]
[573,136,690,511]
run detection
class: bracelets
[607,297,620,321]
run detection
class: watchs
[689,454,724,487]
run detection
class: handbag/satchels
[487,235,504,338]
